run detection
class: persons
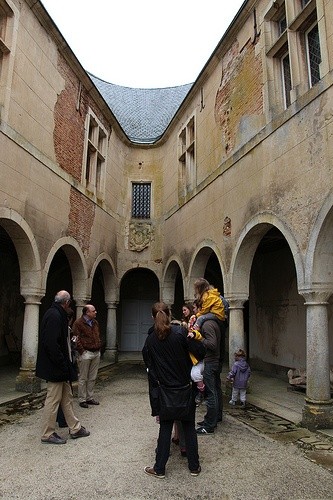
[141,278,230,478]
[35,290,101,444]
[227,349,251,406]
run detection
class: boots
[194,385,208,405]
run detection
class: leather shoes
[79,402,89,408]
[86,399,100,405]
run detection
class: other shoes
[216,418,223,424]
[228,399,235,405]
[181,447,188,457]
[171,436,179,445]
[239,400,246,406]
[57,419,69,428]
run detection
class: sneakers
[190,465,202,476]
[197,422,217,429]
[143,465,166,479]
[40,432,67,444]
[195,427,215,435]
[68,426,90,440]
